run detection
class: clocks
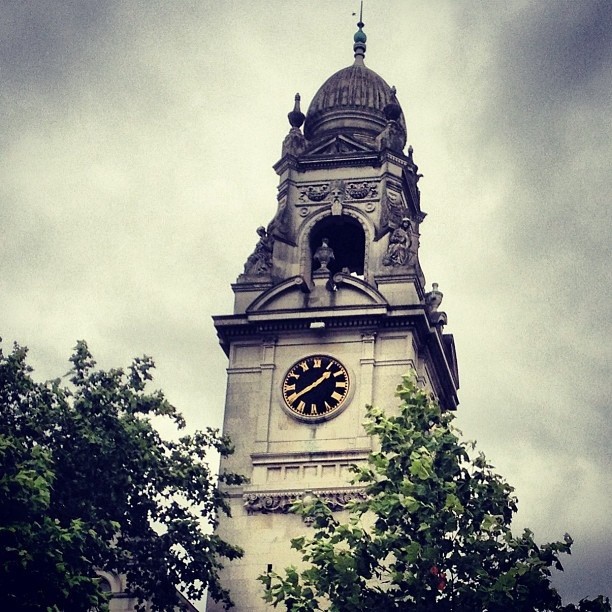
[277,352,356,424]
